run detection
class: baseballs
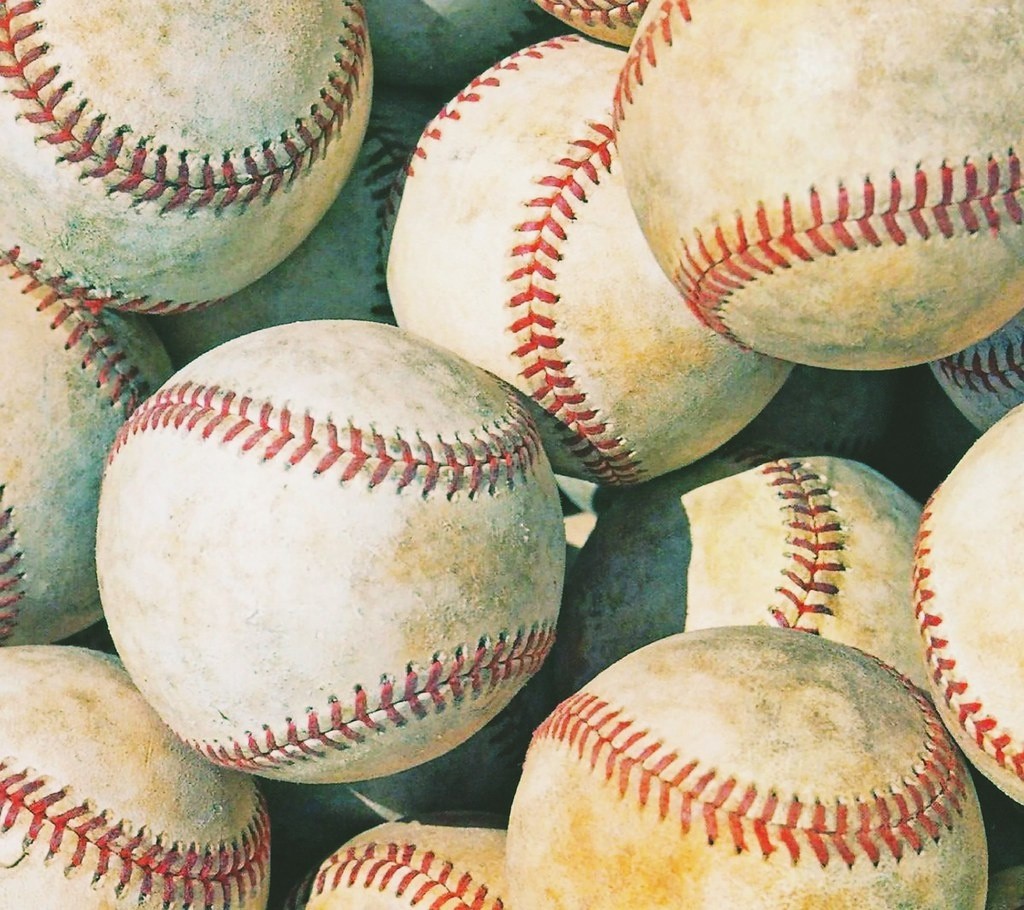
[92,317,568,786]
[1,2,1022,910]
[379,31,794,486]
